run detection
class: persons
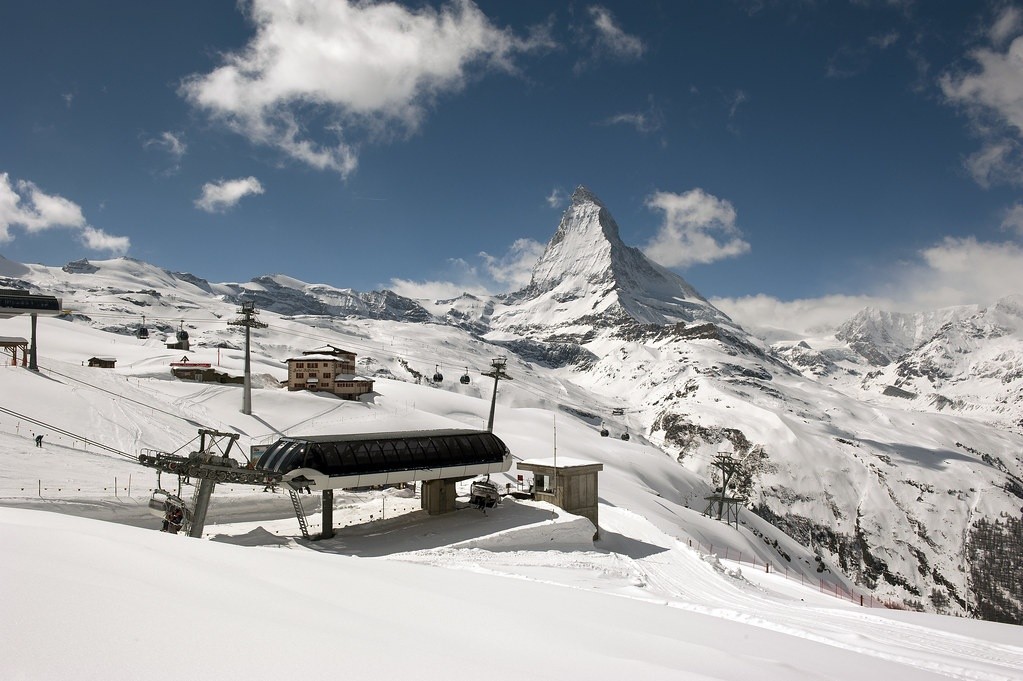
[35,434,44,448]
[478,493,490,510]
[528,477,534,493]
